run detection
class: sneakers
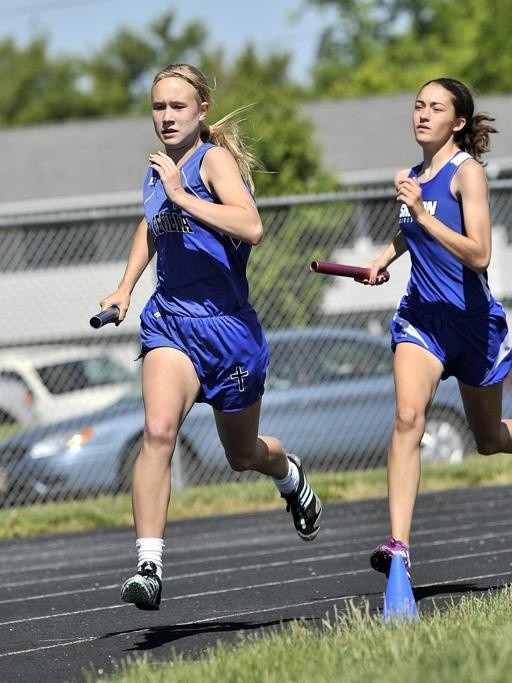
[271,454,322,542]
[370,538,411,579]
[121,561,162,611]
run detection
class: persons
[354,78,512,579]
[99,64,322,610]
